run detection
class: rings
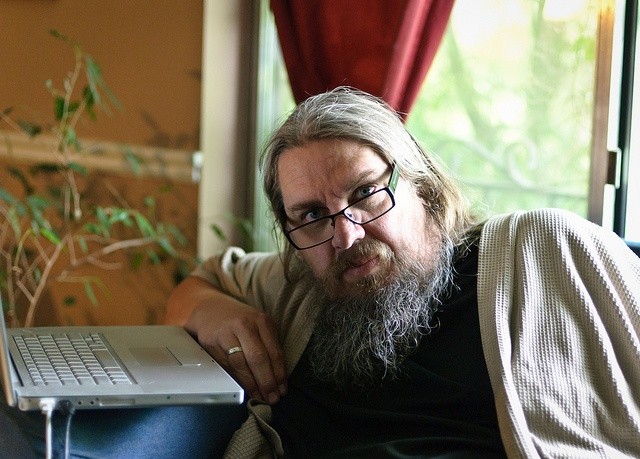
[227,345,243,356]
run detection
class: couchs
[1,237,640,459]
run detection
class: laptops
[0,298,245,413]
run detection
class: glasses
[277,162,399,250]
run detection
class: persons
[163,85,640,458]
